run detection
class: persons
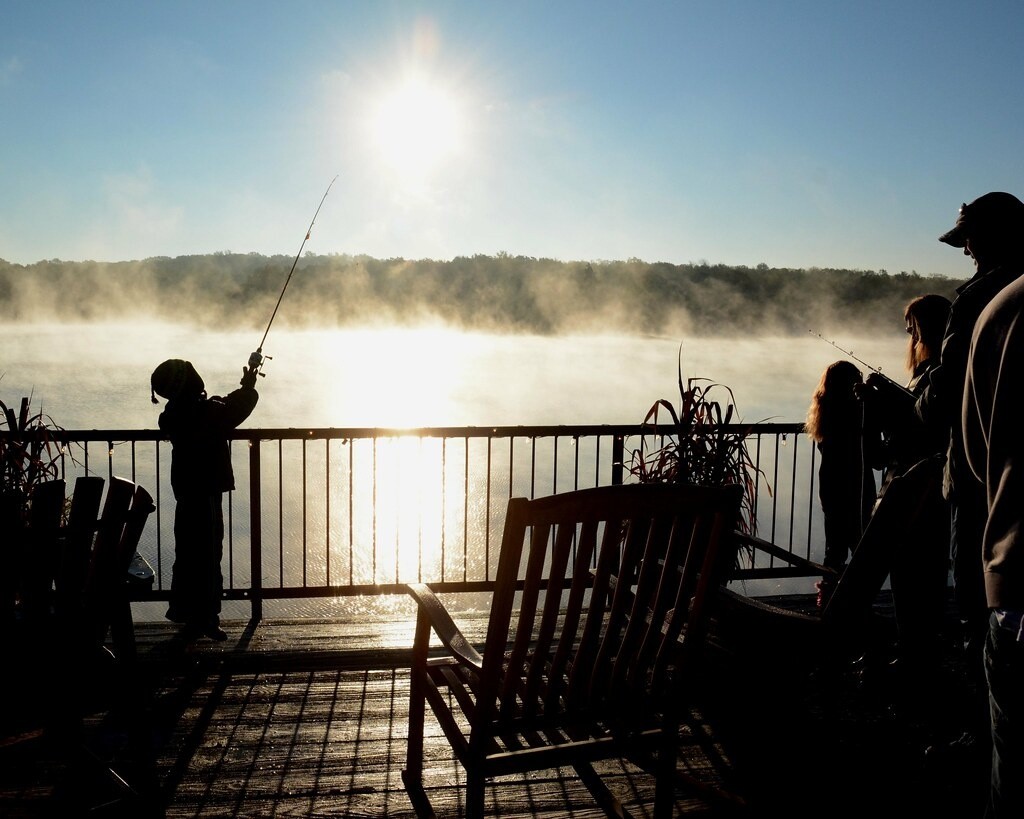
[151,359,259,642]
[814,361,887,606]
[904,192,1024,819]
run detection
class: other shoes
[164,603,229,642]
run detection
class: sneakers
[814,580,839,605]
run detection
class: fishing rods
[237,175,338,383]
[807,327,918,402]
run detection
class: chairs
[0,477,165,819]
[399,483,744,819]
[586,454,943,819]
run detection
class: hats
[937,190,1024,252]
